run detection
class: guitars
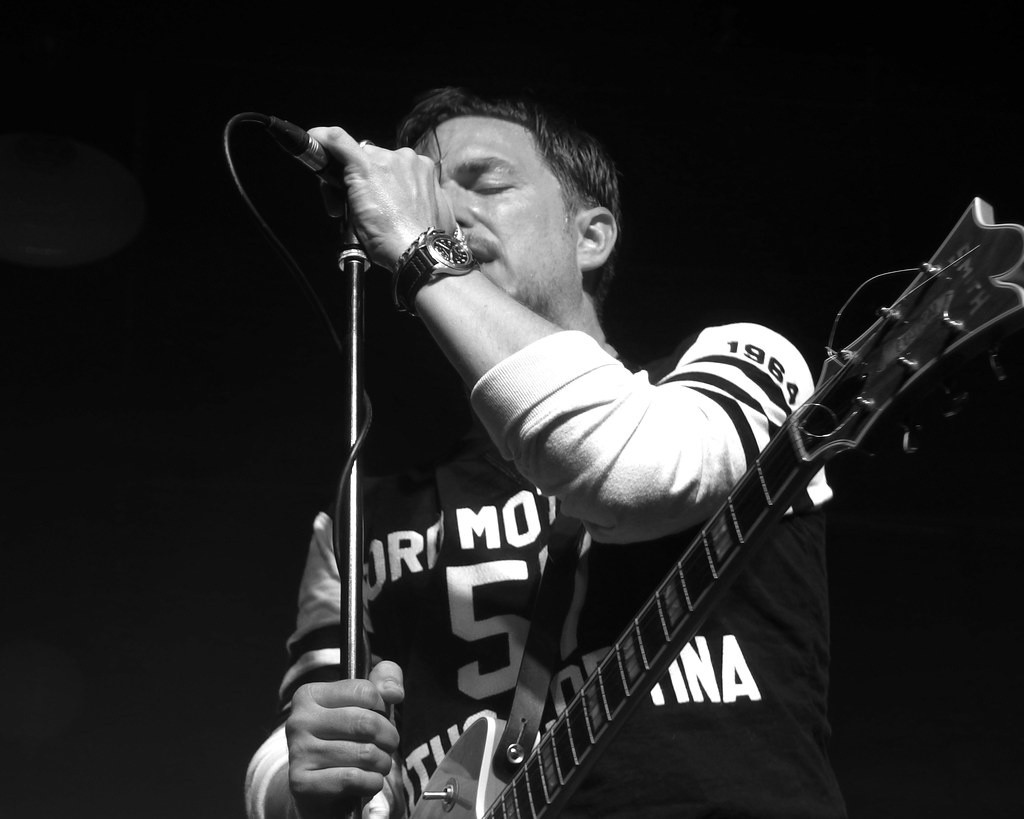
[412,197,1023,819]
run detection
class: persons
[246,88,856,819]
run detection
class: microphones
[266,116,348,194]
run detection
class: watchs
[390,232,483,317]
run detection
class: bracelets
[391,223,447,286]
